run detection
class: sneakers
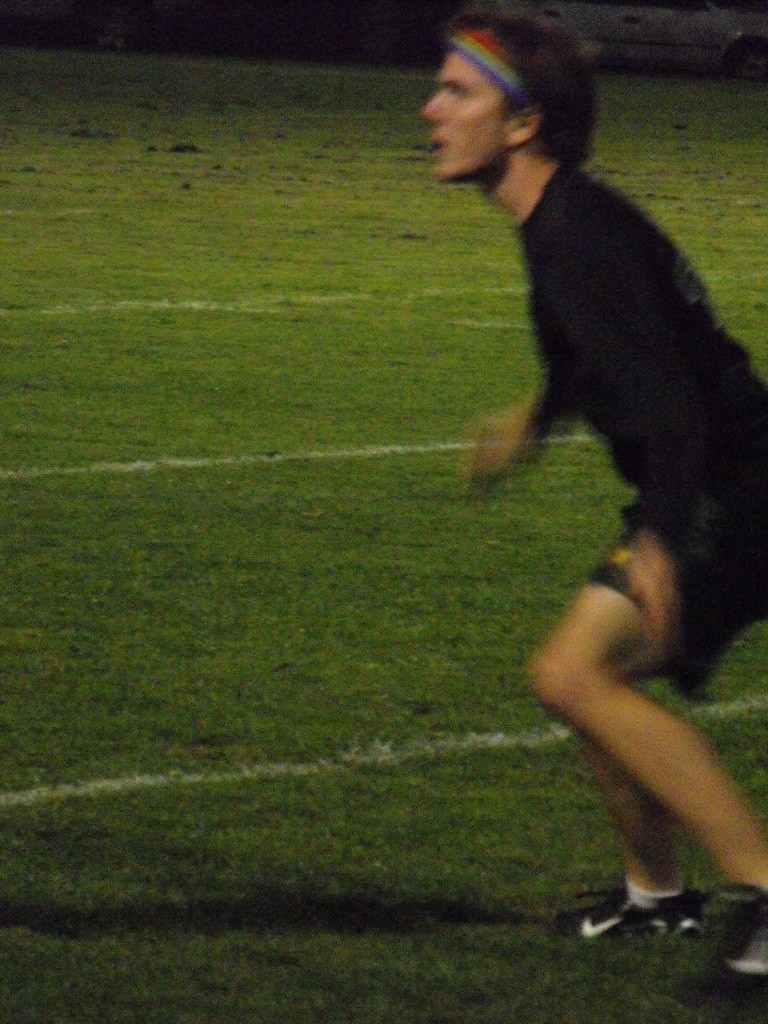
[718,888,768,977]
[552,884,711,942]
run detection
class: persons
[419,7,768,1004]
